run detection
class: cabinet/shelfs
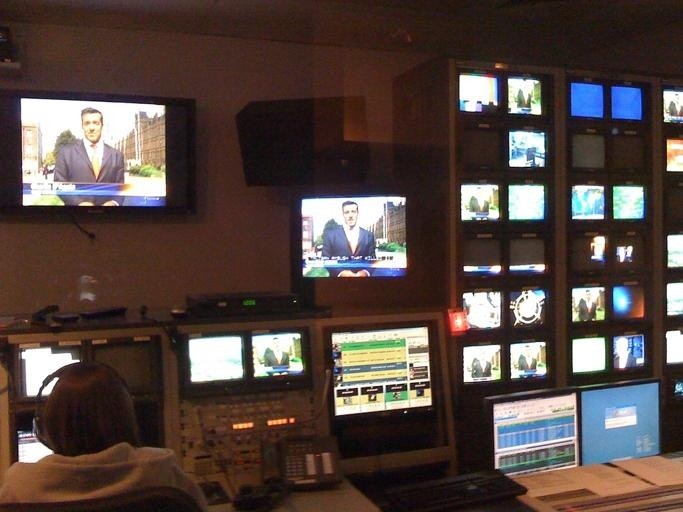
[393,57,559,394]
[659,77,683,448]
[560,66,659,390]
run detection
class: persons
[0,360,209,512]
[263,337,290,377]
[320,201,377,278]
[578,289,597,323]
[667,93,683,123]
[469,187,489,221]
[516,82,532,110]
[52,107,125,207]
[613,337,637,369]
[517,346,537,379]
[470,348,492,383]
[617,246,629,263]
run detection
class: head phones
[32,360,130,450]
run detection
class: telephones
[261,434,345,490]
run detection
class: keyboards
[379,470,527,511]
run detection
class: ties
[90,145,100,176]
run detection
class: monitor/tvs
[0,92,197,221]
[463,234,502,280]
[665,330,683,364]
[289,190,413,282]
[90,344,156,392]
[568,336,608,376]
[249,330,307,391]
[663,135,683,172]
[665,282,683,316]
[506,343,548,380]
[506,233,544,275]
[568,287,604,325]
[610,183,645,222]
[180,331,245,393]
[611,235,642,272]
[459,128,506,165]
[465,291,504,331]
[661,92,683,125]
[610,86,645,123]
[507,289,548,328]
[572,183,606,220]
[482,386,580,474]
[510,130,549,168]
[609,285,645,318]
[582,377,664,469]
[569,81,610,121]
[16,424,54,467]
[504,185,547,220]
[569,232,608,272]
[457,68,503,114]
[610,335,648,372]
[460,184,498,223]
[16,342,85,398]
[667,233,683,269]
[461,345,503,383]
[323,324,433,425]
[610,131,644,170]
[668,186,683,221]
[571,132,607,174]
[507,73,546,115]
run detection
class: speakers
[235,95,371,188]
